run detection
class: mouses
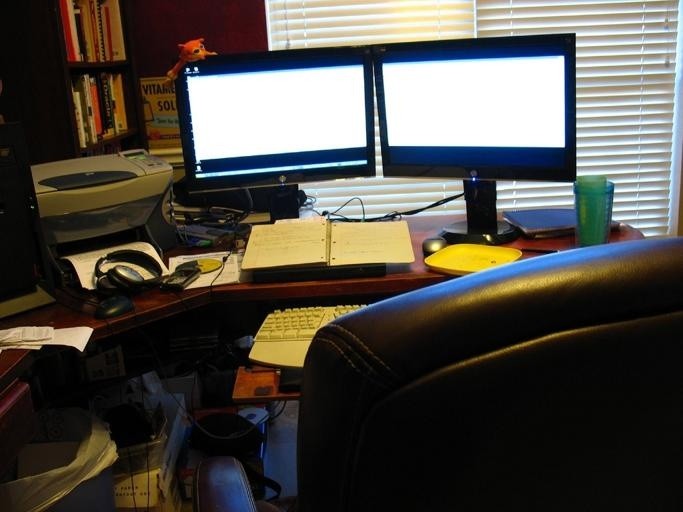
[95,297,136,321]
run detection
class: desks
[0,212,645,425]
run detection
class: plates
[424,243,523,277]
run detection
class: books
[58,0,131,158]
[503,206,622,242]
[242,220,415,274]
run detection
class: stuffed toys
[168,37,217,80]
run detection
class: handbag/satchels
[193,413,255,458]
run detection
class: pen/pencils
[520,248,559,254]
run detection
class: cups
[573,175,614,248]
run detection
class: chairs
[193,235,681,512]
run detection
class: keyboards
[248,300,369,369]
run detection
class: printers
[27,149,184,297]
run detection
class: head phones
[92,250,164,293]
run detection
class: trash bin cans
[1,409,118,511]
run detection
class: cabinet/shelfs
[0,0,148,164]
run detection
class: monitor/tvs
[375,33,577,243]
[173,45,375,222]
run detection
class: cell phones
[166,264,201,289]
